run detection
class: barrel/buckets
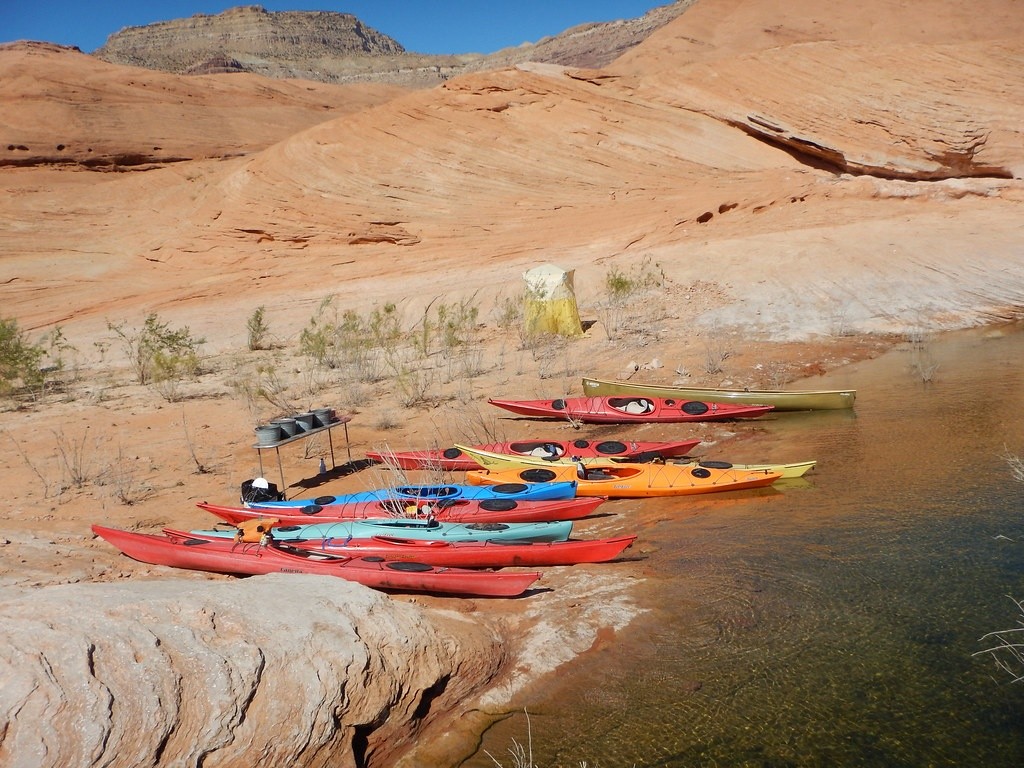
[308,409,331,426]
[286,413,314,432]
[253,424,281,443]
[270,419,297,438]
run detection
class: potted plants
[254,418,281,445]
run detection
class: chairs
[407,515,436,528]
[428,488,450,498]
[625,399,649,414]
[531,443,562,458]
[578,463,612,480]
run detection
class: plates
[252,478,268,489]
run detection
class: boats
[453,444,817,496]
[487,396,776,423]
[582,376,857,410]
[244,480,578,508]
[364,439,701,470]
[91,525,544,596]
[163,527,637,565]
[190,519,573,543]
[196,495,609,528]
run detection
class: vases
[309,408,331,427]
[291,413,315,434]
[270,418,296,438]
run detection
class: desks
[253,418,352,501]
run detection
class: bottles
[320,458,327,474]
